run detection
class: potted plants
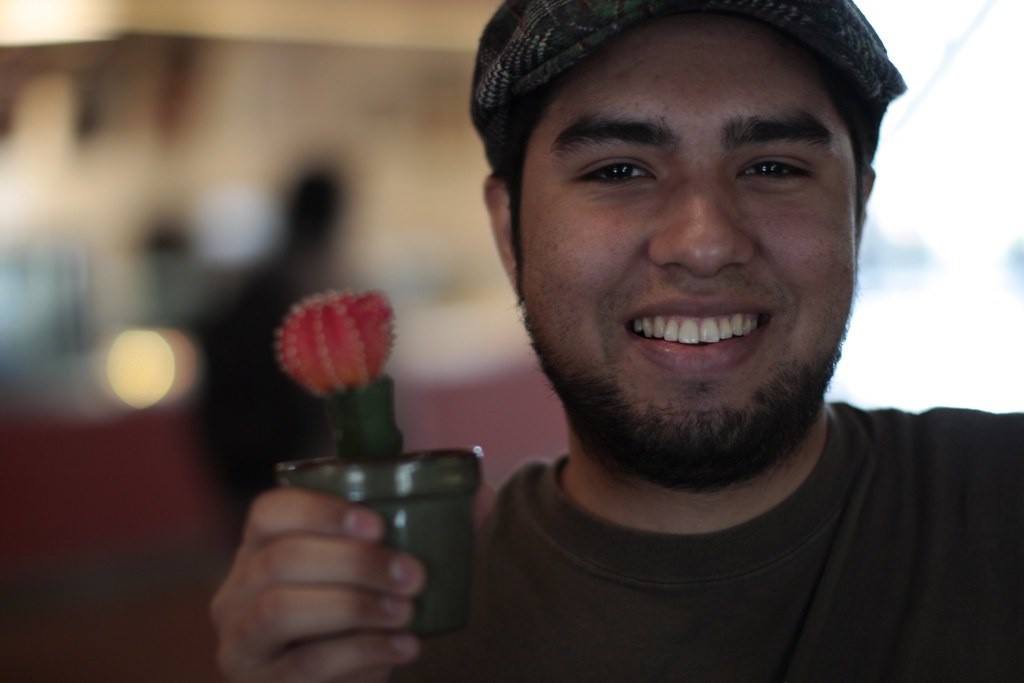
[267,286,496,631]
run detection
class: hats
[469,0,906,168]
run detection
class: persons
[210,0,1024,682]
[197,174,350,541]
